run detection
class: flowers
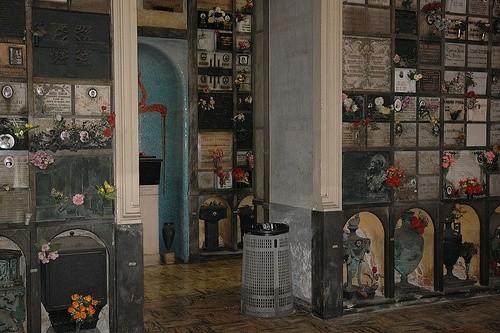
[44,106,116,149]
[51,180,117,206]
[68,294,98,320]
[35,240,60,264]
[195,1,255,187]
[7,118,41,140]
[363,266,383,297]
[31,152,54,169]
[342,2,494,235]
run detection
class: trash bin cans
[241,222,294,317]
